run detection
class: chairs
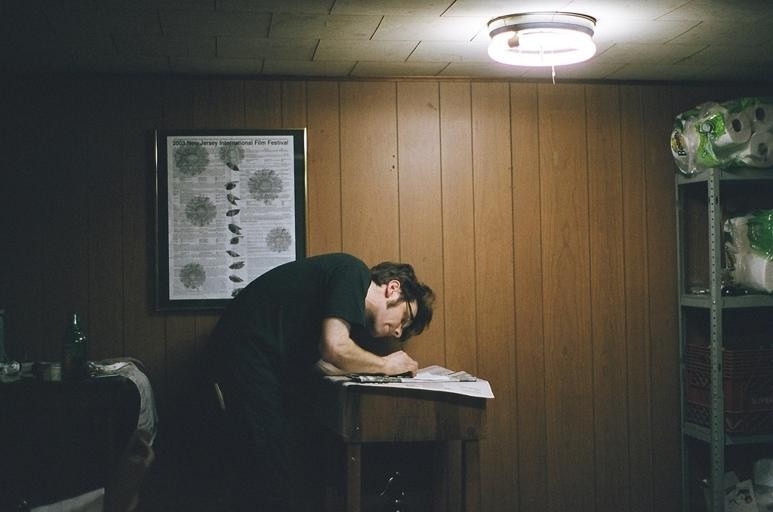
[165,332,250,511]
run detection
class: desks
[318,373,483,511]
[1,355,159,512]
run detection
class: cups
[33,362,62,380]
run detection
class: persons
[207,254,436,512]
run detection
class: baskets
[685,343,773,434]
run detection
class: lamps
[488,12,597,69]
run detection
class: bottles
[63,311,88,378]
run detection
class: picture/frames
[149,125,307,317]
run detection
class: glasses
[401,298,415,329]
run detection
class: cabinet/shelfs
[674,166,772,512]
